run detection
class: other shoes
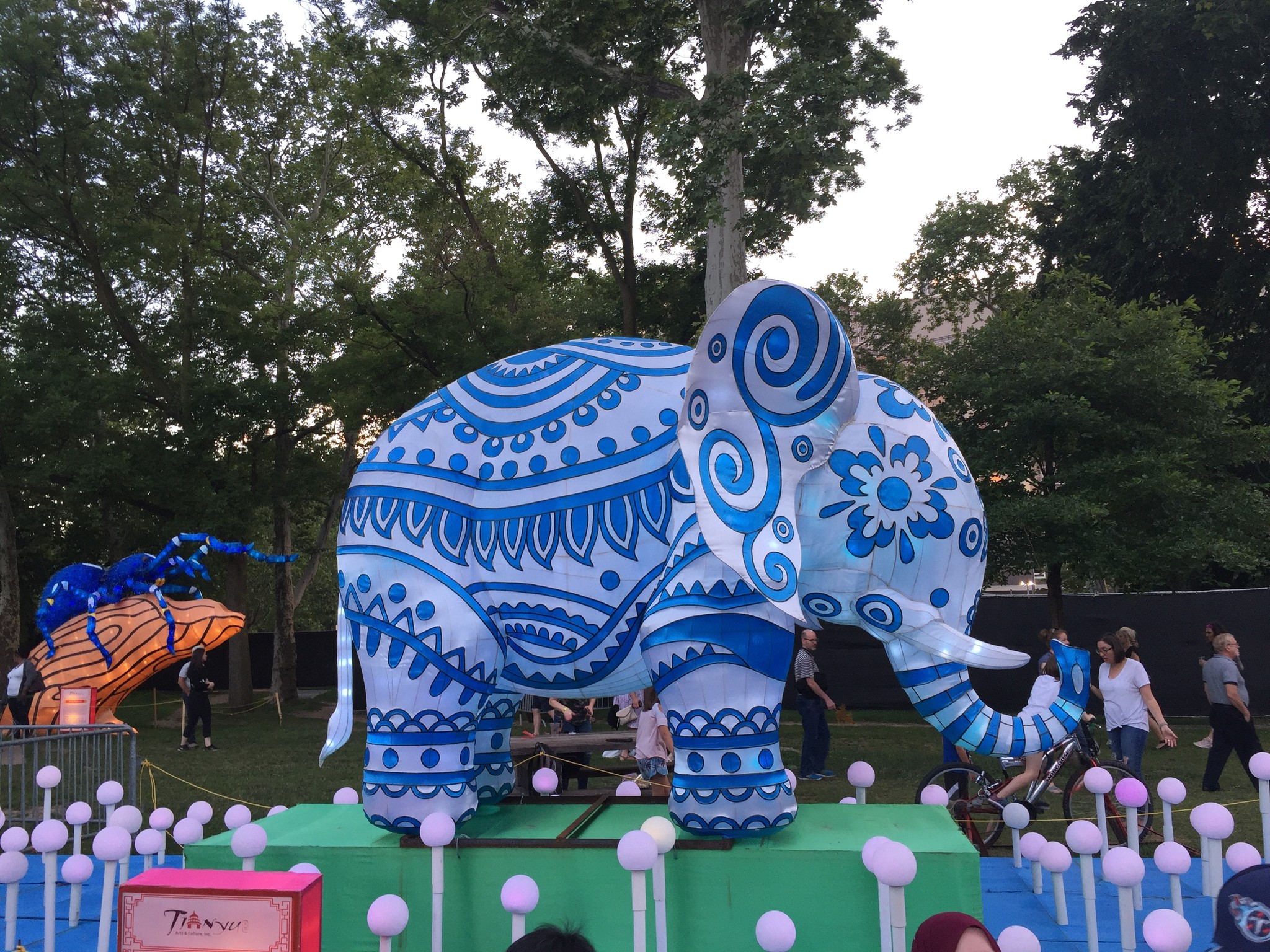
[989,794,1007,810]
[1037,801,1049,809]
[1155,738,1166,749]
[1201,781,1220,792]
[1047,785,1063,793]
[188,743,199,749]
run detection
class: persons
[506,926,596,952]
[635,685,674,797]
[942,735,978,801]
[1191,621,1227,749]
[1118,625,1167,749]
[794,629,837,781]
[531,696,596,790]
[612,690,643,761]
[1038,628,1070,794]
[1201,632,1268,793]
[1087,633,1179,831]
[911,912,1000,952]
[177,646,219,752]
[986,653,1096,810]
[6,649,35,739]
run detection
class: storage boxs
[117,867,323,952]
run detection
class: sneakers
[816,769,836,777]
[799,772,822,781]
[177,745,189,752]
[1193,736,1213,749]
[203,745,220,751]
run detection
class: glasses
[16,648,19,653]
[1228,643,1238,648]
[1097,647,1113,655]
[805,638,819,643]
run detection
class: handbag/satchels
[633,773,653,788]
[607,704,620,730]
[27,672,46,694]
[616,705,638,726]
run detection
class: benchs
[568,761,675,790]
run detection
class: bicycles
[914,715,1154,851]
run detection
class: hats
[192,645,204,653]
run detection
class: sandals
[620,754,636,761]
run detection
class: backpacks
[528,742,565,795]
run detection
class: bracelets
[1158,721,1167,728]
[562,707,568,714]
[1088,684,1093,689]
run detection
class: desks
[510,730,682,792]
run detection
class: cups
[550,722,560,737]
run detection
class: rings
[1168,739,1170,742]
